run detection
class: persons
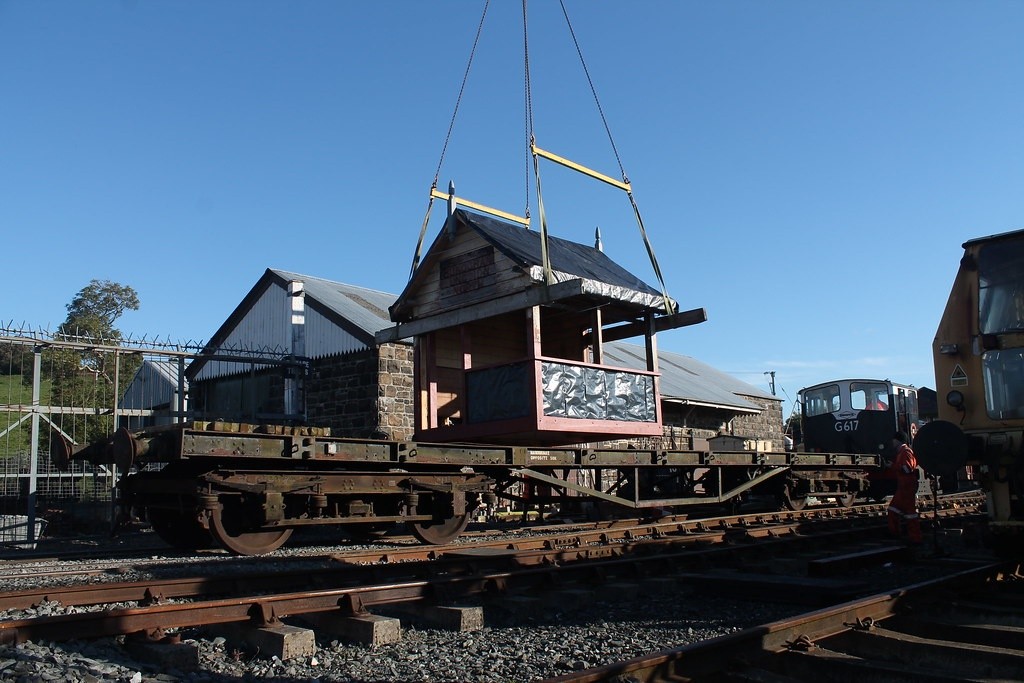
[867,394,887,410]
[883,431,921,546]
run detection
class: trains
[112,378,921,558]
[911,229,1024,552]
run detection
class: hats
[893,431,908,444]
